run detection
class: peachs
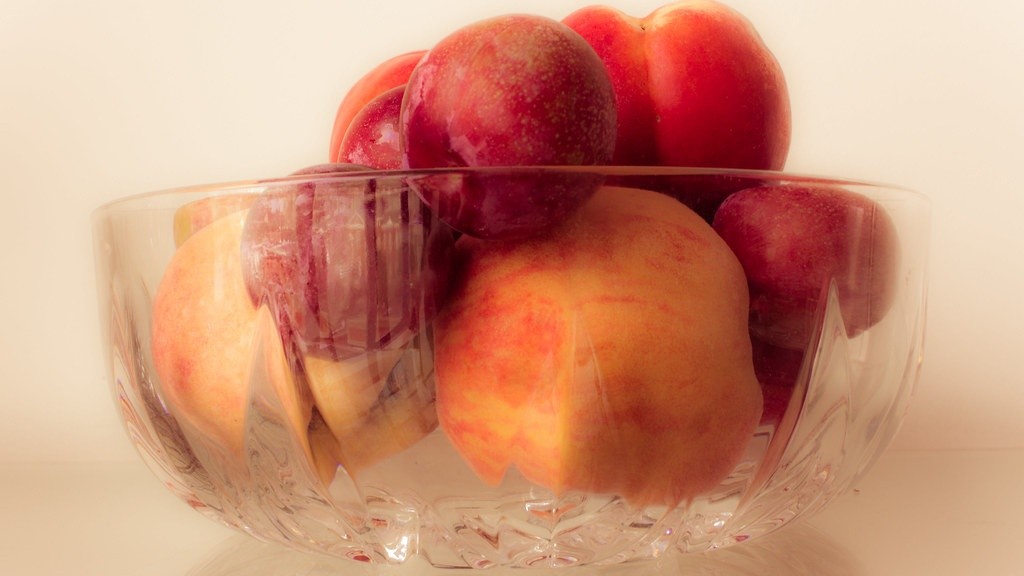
[150,0,904,510]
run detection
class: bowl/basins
[91,164,935,570]
